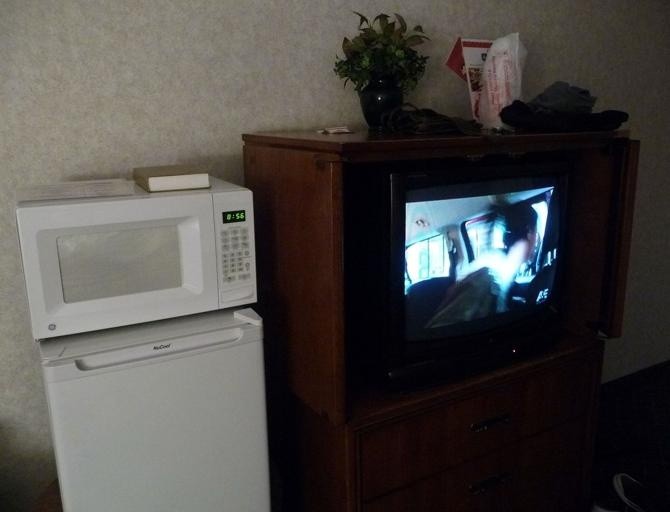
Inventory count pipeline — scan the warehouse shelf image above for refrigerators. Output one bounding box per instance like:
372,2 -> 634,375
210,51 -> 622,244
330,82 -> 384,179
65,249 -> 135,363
33,307 -> 274,512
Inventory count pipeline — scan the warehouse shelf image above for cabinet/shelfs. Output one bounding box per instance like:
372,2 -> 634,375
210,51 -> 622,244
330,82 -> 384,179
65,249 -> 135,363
267,331 -> 607,512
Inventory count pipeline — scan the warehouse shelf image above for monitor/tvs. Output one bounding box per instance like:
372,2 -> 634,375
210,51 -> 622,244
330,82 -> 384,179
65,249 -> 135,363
376,164 -> 570,393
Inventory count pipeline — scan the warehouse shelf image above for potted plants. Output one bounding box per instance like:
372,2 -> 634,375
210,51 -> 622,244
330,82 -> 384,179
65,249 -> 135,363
331,9 -> 430,134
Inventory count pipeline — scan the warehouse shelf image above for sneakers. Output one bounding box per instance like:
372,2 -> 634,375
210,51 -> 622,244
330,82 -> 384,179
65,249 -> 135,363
594,473 -> 645,512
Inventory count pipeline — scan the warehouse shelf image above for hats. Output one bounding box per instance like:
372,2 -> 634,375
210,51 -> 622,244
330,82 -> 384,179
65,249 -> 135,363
498,81 -> 629,131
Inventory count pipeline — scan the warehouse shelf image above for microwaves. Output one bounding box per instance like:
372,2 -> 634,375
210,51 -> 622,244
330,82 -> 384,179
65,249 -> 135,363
16,176 -> 257,343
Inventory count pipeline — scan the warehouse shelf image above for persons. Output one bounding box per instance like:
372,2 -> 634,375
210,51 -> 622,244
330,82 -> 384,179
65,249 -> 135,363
425,198 -> 538,329
